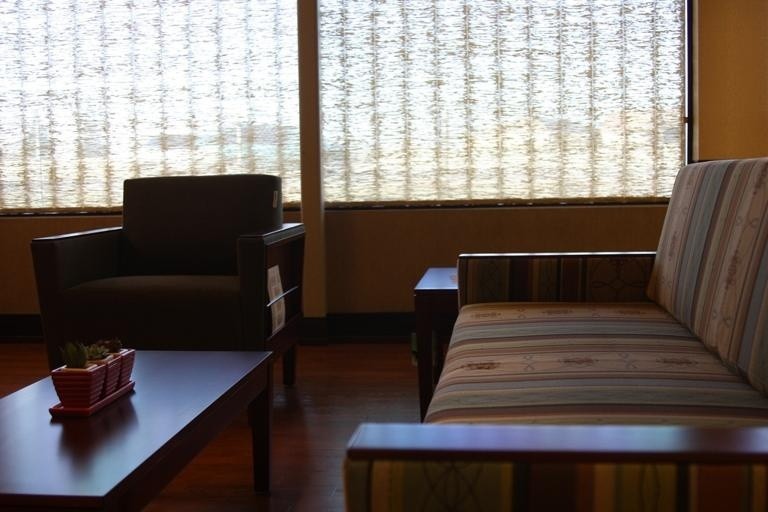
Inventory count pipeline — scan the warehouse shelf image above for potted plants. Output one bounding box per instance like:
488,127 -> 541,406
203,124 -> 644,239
106,337 -> 135,390
51,341 -> 106,406
84,341 -> 122,397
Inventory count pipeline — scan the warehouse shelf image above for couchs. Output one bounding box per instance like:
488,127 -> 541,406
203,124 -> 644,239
343,159 -> 768,511
29,176 -> 307,387
